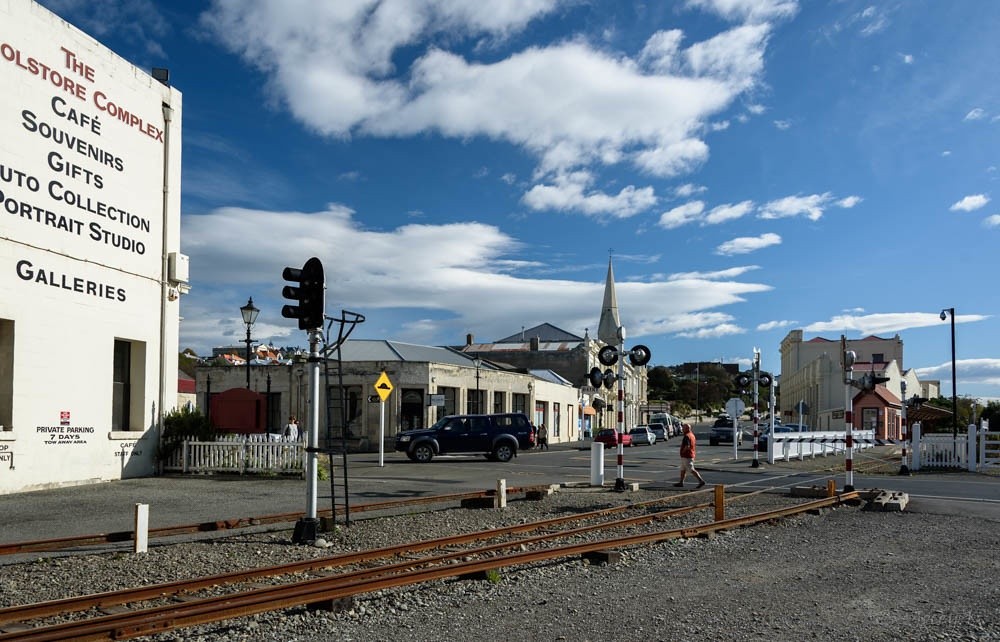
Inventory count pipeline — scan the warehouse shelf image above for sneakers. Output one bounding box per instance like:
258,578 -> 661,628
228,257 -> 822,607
673,481 -> 683,487
696,481 -> 705,489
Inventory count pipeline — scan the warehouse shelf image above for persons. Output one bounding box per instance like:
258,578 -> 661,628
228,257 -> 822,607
531,420 -> 537,437
536,425 -> 542,447
281,415 -> 299,454
293,420 -> 304,455
539,424 -> 550,450
673,423 -> 706,490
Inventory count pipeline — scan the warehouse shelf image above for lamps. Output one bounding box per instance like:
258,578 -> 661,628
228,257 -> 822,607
152,68 -> 169,86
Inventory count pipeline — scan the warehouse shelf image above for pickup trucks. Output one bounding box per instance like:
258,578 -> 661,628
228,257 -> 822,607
595,428 -> 633,448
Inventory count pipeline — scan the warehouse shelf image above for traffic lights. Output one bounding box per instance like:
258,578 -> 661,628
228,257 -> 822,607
280,266 -> 306,320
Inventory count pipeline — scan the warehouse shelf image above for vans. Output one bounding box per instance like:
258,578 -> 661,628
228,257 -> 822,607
649,413 -> 674,437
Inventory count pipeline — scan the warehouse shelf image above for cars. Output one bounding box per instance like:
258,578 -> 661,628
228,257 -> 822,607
709,417 -> 744,446
758,416 -> 808,449
670,415 -> 685,436
392,413 -> 535,463
628,427 -> 657,446
640,423 -> 669,441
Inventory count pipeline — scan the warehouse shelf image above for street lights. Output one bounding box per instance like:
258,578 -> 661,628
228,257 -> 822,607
238,294 -> 261,388
470,352 -> 484,415
940,306 -> 960,466
